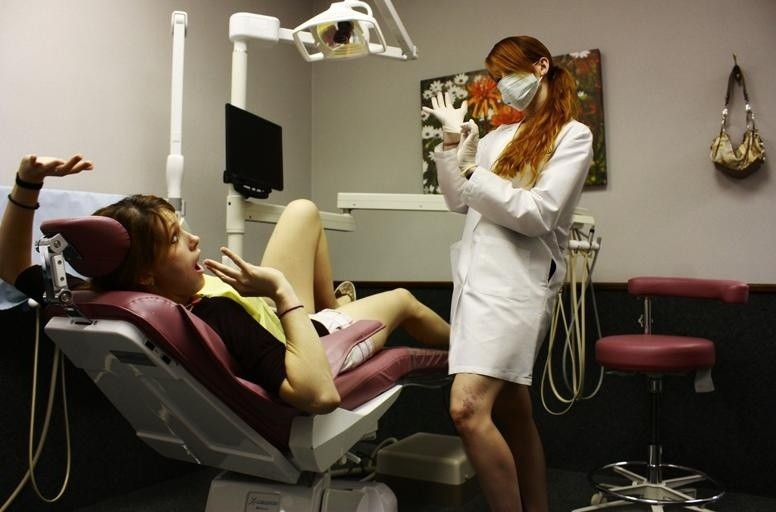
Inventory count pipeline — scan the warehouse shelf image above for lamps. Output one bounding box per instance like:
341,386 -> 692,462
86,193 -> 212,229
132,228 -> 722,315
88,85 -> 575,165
289,0 -> 420,67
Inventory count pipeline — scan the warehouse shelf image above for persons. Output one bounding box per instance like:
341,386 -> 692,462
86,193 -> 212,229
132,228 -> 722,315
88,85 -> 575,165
419,34 -> 596,511
0,154 -> 451,416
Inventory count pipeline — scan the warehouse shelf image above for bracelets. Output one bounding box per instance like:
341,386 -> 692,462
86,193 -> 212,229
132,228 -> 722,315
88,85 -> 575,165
14,172 -> 43,190
6,193 -> 39,212
275,303 -> 304,319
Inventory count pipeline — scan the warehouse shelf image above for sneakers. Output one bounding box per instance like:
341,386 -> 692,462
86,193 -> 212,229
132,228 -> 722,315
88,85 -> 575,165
335,281 -> 356,307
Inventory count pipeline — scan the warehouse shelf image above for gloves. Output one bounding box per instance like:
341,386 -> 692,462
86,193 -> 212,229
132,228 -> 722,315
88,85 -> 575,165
423,92 -> 469,132
458,119 -> 479,174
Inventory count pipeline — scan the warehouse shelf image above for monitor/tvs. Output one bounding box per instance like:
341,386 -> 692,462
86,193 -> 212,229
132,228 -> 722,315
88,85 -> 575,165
224,102 -> 284,200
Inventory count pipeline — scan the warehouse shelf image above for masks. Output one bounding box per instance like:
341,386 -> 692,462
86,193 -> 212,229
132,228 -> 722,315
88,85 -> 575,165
496,63 -> 543,111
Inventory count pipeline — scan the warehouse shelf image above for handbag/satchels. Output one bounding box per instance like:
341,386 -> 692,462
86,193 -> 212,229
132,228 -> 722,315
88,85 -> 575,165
709,129 -> 766,178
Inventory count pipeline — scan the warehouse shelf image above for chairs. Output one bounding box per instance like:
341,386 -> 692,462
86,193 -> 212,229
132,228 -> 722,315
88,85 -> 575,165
570,275 -> 750,512
33,215 -> 451,512
